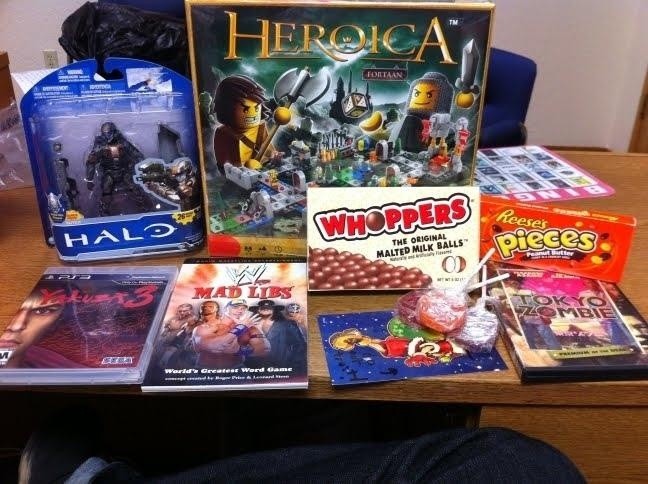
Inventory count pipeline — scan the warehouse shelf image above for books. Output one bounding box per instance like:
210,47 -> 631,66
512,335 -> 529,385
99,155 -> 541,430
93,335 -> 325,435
485,259 -> 648,384
0,266 -> 180,386
139,255 -> 308,392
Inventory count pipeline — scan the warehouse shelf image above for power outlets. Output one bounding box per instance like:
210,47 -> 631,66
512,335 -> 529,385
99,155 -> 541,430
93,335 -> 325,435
42,50 -> 59,69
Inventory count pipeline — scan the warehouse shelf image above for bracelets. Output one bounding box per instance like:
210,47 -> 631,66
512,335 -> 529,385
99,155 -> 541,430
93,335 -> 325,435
227,323 -> 248,338
233,344 -> 254,357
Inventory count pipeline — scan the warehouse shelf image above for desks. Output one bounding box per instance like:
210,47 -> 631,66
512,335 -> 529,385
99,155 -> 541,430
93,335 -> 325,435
0,149 -> 647,484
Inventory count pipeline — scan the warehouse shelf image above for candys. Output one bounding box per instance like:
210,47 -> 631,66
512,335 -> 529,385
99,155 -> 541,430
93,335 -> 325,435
396,246 -> 512,354
310,243 -> 431,291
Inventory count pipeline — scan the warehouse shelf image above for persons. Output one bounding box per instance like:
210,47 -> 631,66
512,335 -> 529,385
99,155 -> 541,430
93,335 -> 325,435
173,300 -> 222,366
192,297 -> 271,370
18,425 -> 588,484
152,303 -> 198,370
249,299 -> 307,367
1,285 -> 92,368
287,303 -> 307,340
